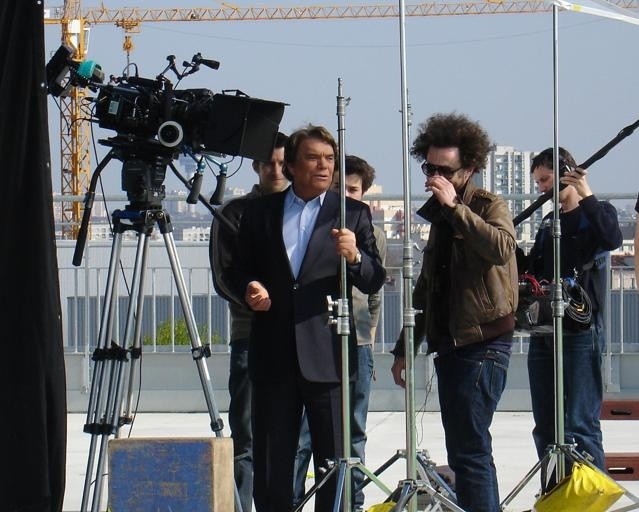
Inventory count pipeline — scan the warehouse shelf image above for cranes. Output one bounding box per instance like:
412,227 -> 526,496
44,0 -> 567,241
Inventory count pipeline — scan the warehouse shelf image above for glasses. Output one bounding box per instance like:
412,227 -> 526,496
421,160 -> 466,180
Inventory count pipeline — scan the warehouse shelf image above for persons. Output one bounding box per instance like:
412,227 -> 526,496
510,144 -> 623,497
388,108 -> 521,512
222,125 -> 385,511
288,156 -> 388,511
209,128 -> 291,512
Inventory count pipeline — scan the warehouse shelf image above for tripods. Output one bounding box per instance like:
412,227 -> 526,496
79,209 -> 243,512
293,78 -> 406,512
355,0 -> 465,512
500,1 -> 639,512
